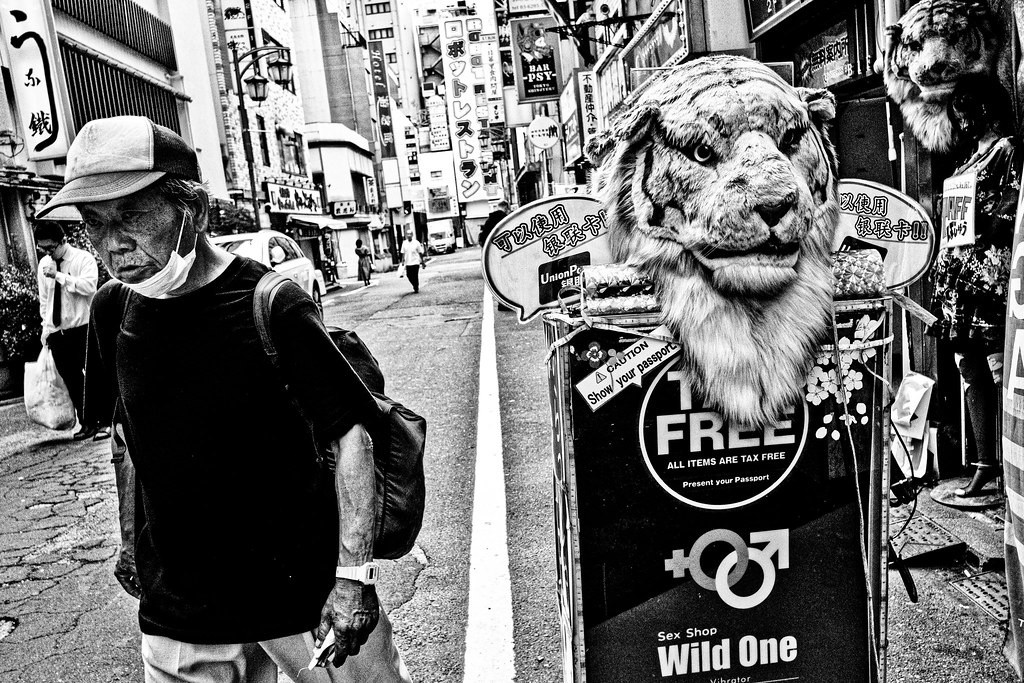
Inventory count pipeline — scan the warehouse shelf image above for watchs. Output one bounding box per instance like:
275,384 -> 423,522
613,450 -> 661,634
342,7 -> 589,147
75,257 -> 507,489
336,562 -> 379,585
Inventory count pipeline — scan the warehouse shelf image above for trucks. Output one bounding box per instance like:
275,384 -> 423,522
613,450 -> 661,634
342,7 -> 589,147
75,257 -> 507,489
426,216 -> 457,256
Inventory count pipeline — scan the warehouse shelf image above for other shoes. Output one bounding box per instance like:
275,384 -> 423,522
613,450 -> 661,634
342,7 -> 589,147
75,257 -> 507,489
73,425 -> 112,441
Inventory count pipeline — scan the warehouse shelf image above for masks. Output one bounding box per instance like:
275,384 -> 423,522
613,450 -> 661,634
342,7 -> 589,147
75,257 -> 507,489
105,210 -> 198,301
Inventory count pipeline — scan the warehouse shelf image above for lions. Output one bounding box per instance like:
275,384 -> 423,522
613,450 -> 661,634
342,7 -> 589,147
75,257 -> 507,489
584,53 -> 842,435
882,0 -> 1006,154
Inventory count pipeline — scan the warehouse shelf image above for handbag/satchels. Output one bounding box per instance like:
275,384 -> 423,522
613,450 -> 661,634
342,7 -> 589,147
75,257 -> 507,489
24,344 -> 77,432
397,263 -> 406,279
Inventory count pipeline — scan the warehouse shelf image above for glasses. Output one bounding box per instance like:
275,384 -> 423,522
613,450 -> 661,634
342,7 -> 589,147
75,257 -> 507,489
36,242 -> 61,253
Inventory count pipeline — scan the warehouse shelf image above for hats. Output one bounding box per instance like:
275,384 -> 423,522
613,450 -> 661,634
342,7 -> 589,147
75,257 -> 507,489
35,114 -> 201,218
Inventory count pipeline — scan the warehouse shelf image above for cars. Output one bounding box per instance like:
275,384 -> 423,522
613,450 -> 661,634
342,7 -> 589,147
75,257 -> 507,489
209,229 -> 327,322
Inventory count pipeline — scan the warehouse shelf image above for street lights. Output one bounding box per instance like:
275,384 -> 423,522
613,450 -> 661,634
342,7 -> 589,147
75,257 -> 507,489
228,35 -> 293,232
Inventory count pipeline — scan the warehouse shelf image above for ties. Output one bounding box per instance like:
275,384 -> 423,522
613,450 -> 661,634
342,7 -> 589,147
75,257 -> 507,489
52,257 -> 64,327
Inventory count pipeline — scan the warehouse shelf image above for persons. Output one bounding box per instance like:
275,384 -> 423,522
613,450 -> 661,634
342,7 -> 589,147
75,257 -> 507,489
924,86 -> 1021,498
400,230 -> 426,294
35,115 -> 413,683
34,222 -> 113,442
355,238 -> 373,286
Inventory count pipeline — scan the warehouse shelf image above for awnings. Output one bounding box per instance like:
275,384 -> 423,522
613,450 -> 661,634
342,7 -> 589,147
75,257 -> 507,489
290,215 -> 347,230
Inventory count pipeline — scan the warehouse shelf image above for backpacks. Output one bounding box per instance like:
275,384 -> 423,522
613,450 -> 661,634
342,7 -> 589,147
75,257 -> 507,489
113,269 -> 426,561
